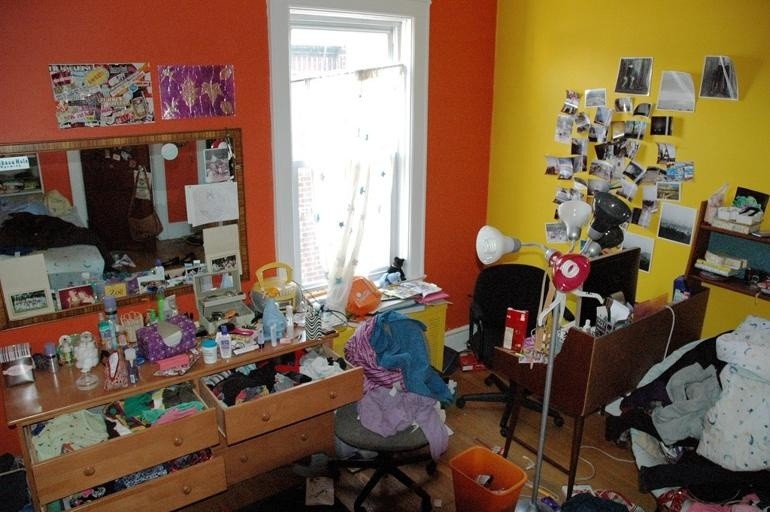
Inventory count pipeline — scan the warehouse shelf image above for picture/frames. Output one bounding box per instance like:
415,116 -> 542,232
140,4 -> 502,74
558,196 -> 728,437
1,152 -> 44,195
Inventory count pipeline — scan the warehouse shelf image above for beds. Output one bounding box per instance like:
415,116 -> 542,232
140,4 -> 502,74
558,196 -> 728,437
0,195 -> 105,289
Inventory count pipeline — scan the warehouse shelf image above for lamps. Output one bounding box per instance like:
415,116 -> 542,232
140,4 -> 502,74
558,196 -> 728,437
546,248 -> 589,292
476,225 -> 547,264
161,142 -> 187,161
589,226 -> 624,256
559,200 -> 593,255
587,191 -> 632,240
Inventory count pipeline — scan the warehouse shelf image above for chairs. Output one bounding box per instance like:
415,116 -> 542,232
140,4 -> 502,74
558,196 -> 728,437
457,263 -> 563,437
324,312 -> 438,512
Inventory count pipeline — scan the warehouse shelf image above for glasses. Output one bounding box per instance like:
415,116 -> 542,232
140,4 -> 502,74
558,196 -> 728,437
231,341 -> 245,351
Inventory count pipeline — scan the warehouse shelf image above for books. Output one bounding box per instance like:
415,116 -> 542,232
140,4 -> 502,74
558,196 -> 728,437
695,249 -> 747,278
711,218 -> 760,236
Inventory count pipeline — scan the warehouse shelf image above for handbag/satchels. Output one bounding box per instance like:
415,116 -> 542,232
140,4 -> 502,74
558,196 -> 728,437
126,164 -> 164,242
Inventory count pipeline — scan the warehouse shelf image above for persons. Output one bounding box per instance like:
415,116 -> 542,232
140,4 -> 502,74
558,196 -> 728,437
143,281 -> 158,293
67,289 -> 80,308
79,291 -> 94,304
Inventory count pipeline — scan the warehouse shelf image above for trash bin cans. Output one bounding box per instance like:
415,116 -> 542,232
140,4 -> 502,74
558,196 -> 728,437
449,445 -> 527,512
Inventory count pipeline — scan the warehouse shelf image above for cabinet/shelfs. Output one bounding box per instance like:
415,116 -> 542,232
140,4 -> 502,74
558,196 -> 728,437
7,332 -> 363,512
682,200 -> 770,337
310,301 -> 446,374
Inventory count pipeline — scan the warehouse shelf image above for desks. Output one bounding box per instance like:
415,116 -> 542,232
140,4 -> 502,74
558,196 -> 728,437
492,279 -> 710,501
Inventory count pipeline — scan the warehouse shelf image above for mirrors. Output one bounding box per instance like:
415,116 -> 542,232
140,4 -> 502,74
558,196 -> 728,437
0,128 -> 251,333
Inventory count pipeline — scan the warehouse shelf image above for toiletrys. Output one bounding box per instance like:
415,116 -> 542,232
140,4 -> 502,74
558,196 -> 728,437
200,340 -> 217,364
257,326 -> 265,349
233,344 -> 259,355
286,306 -> 294,338
271,324 -> 277,347
219,325 -> 232,359
